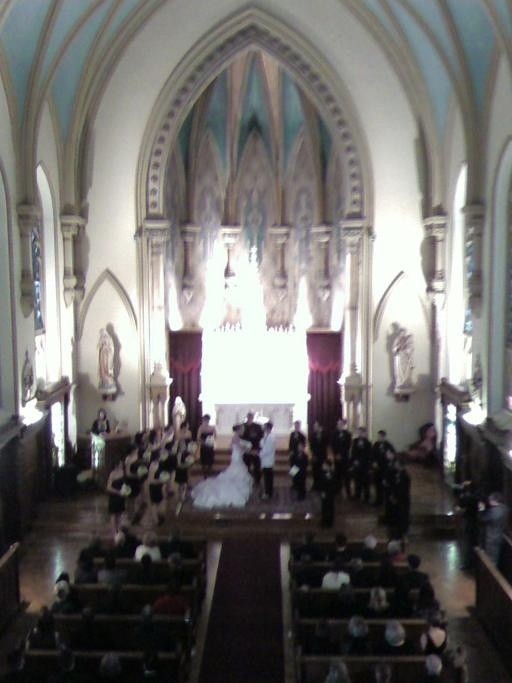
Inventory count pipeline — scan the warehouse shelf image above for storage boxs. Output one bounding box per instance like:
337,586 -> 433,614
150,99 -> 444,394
288,534 -> 469,682
22,537 -> 206,683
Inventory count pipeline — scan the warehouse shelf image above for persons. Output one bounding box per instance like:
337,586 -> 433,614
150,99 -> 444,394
308,420 -> 329,490
10,527 -> 197,681
259,423 -> 275,499
90,410 -> 111,436
96,328 -> 115,389
392,328 -> 417,391
107,420 -> 201,535
240,413 -> 265,488
373,431 -> 411,534
190,424 -> 254,510
291,531 -> 468,681
319,459 -> 341,528
197,415 -> 217,480
289,441 -> 308,501
288,421 -> 306,488
351,428 -> 373,503
330,419 -> 352,499
